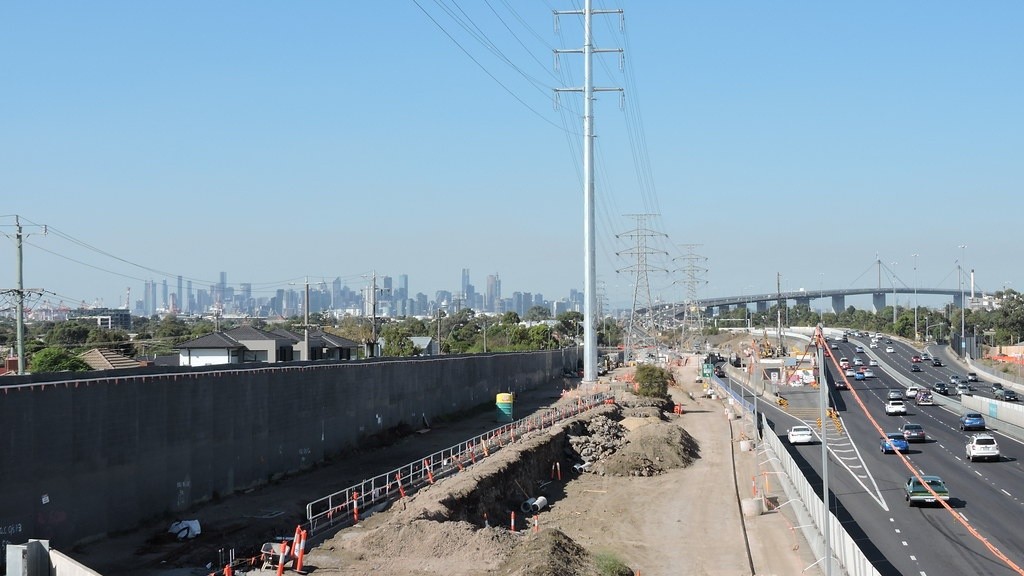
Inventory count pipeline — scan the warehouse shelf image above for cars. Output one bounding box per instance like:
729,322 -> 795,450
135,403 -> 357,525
931,358 -> 941,367
819,329 -> 896,381
884,400 -> 907,416
959,411 -> 986,431
786,425 -> 814,445
880,432 -> 910,455
887,389 -> 903,401
906,387 -> 919,399
920,352 -> 929,360
900,423 -> 925,443
910,364 -> 920,373
916,389 -> 934,406
911,355 -> 921,363
949,371 -> 1019,403
903,475 -> 950,508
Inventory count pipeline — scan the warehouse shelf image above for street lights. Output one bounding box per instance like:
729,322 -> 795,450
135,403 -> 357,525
957,245 -> 969,337
891,261 -> 899,325
819,273 -> 825,320
912,254 -> 920,342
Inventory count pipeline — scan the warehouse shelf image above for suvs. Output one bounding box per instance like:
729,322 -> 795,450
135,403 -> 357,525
964,432 -> 1001,463
933,382 -> 949,396
